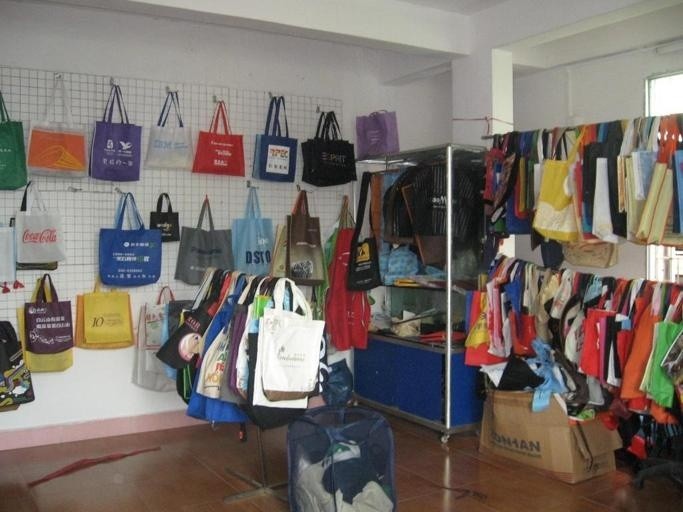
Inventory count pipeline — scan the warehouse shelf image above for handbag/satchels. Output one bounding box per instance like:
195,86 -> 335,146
0,212 -> 73,412
174,196 -> 234,285
464,275 -> 682,425
1,91 -> 28,190
150,192 -> 180,243
191,101 -> 245,177
269,223 -> 287,277
143,93 -> 194,170
561,244 -> 617,268
28,75 -> 86,172
252,96 -> 297,182
74,291 -> 134,350
131,296 -> 327,430
99,192 -> 162,286
327,195 -> 381,291
355,112 -> 396,160
471,143 -> 681,247
230,189 -> 274,277
286,192 -> 326,286
88,84 -> 142,182
300,112 -> 358,187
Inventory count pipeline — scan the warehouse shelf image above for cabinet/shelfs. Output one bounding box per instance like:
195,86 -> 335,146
348,143 -> 491,444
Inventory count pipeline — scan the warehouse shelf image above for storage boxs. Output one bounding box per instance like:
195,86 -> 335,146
479,375 -> 625,485
